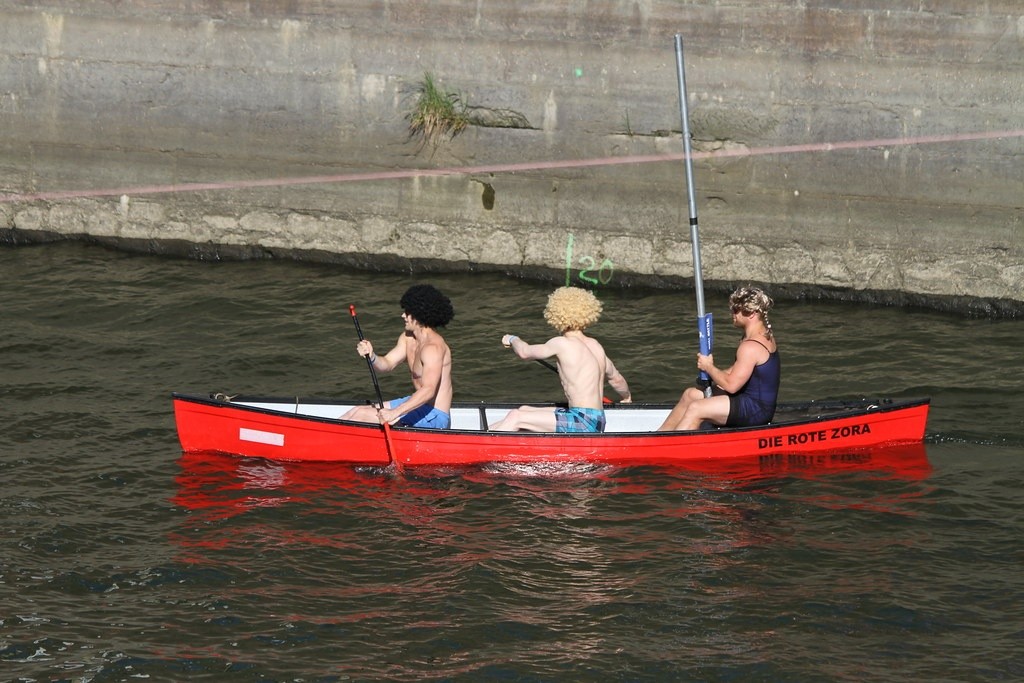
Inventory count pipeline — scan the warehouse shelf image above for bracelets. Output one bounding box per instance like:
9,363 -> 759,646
372,355 -> 377,363
509,336 -> 518,344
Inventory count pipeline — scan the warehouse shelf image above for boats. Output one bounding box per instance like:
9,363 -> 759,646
172,392 -> 932,466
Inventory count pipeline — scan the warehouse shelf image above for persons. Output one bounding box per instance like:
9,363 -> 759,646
336,284 -> 456,429
489,285 -> 631,432
657,286 -> 780,431
